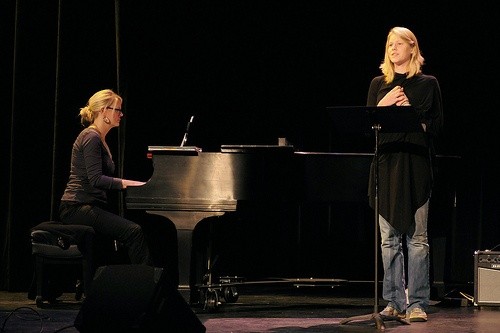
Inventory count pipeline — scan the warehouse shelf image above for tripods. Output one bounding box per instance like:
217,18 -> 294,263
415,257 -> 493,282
325,104 -> 417,331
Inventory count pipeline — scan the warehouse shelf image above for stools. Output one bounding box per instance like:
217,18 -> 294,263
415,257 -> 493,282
31,242 -> 87,310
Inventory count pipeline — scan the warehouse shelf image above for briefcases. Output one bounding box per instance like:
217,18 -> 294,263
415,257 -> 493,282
473,244 -> 500,307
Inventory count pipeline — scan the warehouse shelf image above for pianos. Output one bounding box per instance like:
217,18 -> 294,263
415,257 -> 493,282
122,143 -> 298,311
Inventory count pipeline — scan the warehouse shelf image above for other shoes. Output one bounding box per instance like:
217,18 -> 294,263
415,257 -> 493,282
408,307 -> 428,322
379,306 -> 407,318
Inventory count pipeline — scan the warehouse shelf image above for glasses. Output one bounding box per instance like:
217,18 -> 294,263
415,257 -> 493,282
106,106 -> 122,116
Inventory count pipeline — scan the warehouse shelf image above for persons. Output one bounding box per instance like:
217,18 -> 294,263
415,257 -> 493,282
367,26 -> 442,322
60,89 -> 180,290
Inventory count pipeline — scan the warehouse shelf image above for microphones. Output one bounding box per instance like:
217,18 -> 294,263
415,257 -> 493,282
181,114 -> 193,147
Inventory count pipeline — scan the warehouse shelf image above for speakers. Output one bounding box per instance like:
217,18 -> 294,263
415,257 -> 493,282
72,263 -> 206,333
473,251 -> 500,310
